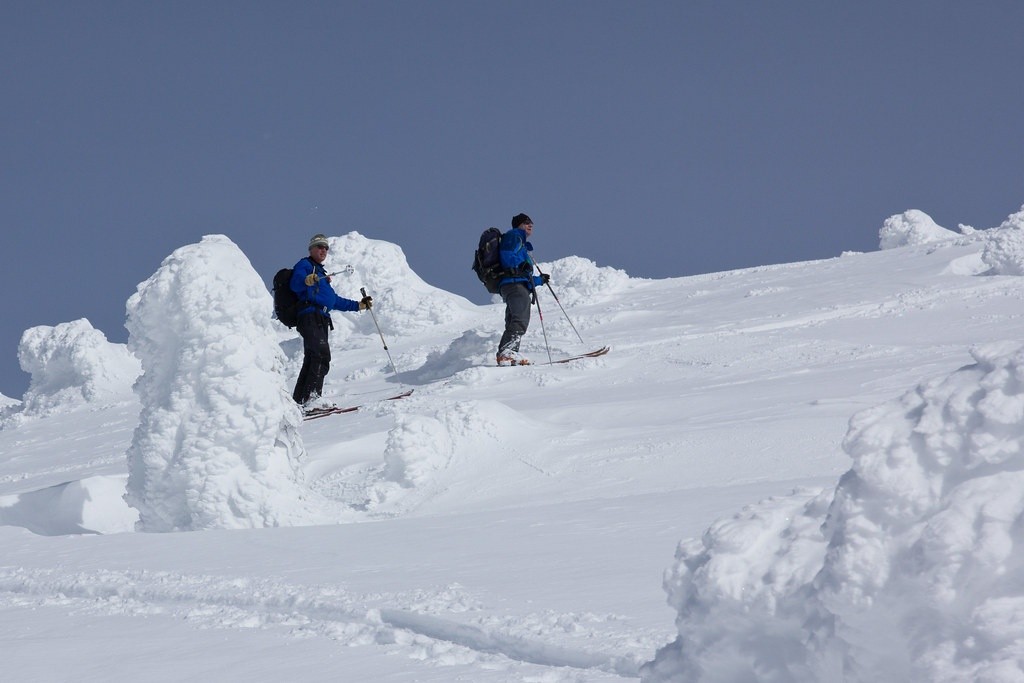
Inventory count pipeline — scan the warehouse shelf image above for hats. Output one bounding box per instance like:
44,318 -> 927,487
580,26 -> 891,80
512,213 -> 529,229
308,233 -> 328,250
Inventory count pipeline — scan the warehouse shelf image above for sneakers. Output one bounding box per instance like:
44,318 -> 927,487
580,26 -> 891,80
496,351 -> 531,366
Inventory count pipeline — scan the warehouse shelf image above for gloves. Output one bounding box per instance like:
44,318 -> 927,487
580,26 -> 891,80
540,274 -> 550,286
358,296 -> 373,310
518,261 -> 533,274
304,274 -> 319,286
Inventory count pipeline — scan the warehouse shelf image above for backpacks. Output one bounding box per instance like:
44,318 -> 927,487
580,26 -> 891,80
272,268 -> 296,329
472,227 -> 501,294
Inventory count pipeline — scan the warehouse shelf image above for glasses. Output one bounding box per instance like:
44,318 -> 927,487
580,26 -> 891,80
318,245 -> 329,250
522,219 -> 534,224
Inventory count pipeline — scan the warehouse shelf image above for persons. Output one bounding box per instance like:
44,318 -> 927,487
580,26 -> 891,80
291,234 -> 373,412
497,213 -> 550,366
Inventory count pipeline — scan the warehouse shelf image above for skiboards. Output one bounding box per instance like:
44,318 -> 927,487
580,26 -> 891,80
303,388 -> 414,422
477,344 -> 612,367
